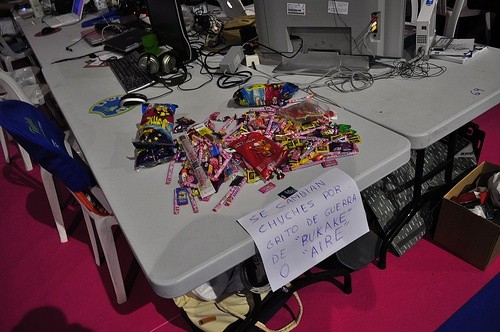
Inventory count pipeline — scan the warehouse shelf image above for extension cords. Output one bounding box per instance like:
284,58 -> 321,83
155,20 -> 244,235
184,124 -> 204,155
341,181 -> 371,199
243,43 -> 260,66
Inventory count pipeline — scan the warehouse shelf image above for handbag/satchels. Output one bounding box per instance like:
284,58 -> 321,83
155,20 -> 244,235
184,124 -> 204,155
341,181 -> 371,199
223,15 -> 259,47
173,257 -> 302,332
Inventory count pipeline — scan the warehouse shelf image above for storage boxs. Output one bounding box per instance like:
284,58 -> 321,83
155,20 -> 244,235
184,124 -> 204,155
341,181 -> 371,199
435,160 -> 500,272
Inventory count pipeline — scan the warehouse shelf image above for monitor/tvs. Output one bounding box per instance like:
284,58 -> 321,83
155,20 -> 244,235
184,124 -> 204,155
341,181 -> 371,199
144,0 -> 199,66
253,0 -> 406,77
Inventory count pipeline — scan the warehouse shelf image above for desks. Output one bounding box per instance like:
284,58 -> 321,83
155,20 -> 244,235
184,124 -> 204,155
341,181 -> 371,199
10,7 -> 110,67
225,34 -> 500,269
41,45 -> 411,332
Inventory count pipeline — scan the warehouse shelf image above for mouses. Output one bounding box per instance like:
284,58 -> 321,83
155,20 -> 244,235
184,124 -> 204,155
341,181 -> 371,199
119,93 -> 147,106
42,27 -> 55,34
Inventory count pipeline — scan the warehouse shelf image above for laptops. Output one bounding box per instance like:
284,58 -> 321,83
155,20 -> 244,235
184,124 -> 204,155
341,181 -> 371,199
218,0 -> 256,17
42,0 -> 84,28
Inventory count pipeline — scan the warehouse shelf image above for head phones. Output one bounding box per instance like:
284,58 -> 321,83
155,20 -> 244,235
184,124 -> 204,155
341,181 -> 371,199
137,52 -> 187,86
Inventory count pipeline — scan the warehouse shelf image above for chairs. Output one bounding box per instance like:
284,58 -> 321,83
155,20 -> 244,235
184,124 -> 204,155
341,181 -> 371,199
0,37 -> 126,303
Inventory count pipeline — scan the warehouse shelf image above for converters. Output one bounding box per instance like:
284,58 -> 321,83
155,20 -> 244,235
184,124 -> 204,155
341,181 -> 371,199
220,46 -> 244,73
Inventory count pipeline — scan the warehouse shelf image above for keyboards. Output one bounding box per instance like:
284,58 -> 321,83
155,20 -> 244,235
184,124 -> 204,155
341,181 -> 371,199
107,50 -> 162,93
